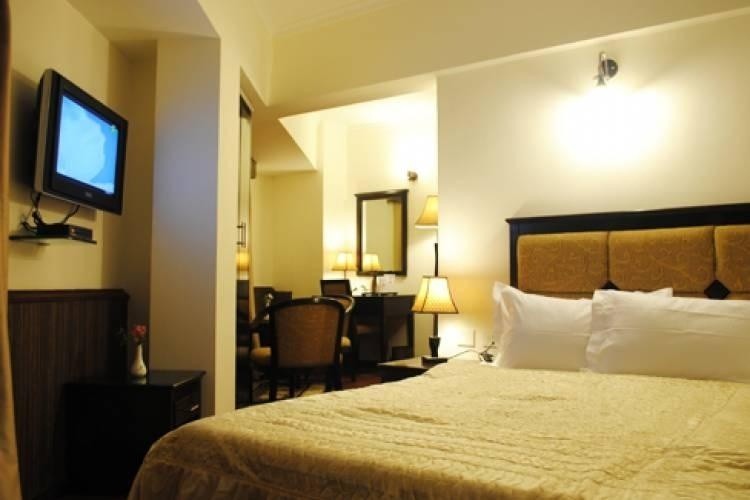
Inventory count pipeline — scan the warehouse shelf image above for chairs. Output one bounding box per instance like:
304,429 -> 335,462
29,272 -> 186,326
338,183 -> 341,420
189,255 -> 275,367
312,294 -> 356,392
244,295 -> 346,408
320,279 -> 380,368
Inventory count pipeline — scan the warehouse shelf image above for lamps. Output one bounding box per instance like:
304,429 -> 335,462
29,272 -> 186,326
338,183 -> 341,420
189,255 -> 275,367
411,241 -> 460,366
363,254 -> 383,270
331,253 -> 357,279
415,196 -> 439,277
591,49 -> 620,88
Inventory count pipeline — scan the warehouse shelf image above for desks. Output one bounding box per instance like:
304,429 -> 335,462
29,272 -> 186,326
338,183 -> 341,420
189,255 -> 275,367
352,294 -> 416,368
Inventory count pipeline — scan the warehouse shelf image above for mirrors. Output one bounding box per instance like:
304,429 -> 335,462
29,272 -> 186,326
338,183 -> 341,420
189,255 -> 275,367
354,189 -> 409,277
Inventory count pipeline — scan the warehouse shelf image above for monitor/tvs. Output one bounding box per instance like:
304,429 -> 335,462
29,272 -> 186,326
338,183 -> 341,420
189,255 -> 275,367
32,69 -> 128,215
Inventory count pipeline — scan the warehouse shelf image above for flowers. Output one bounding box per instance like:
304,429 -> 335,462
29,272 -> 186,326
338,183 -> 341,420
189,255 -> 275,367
118,324 -> 151,348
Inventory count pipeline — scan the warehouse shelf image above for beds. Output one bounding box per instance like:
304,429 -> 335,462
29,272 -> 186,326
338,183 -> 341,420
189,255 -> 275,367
126,200 -> 748,500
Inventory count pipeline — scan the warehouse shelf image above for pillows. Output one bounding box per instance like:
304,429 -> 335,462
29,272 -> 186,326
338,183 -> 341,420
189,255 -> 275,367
585,287 -> 748,383
490,277 -> 673,374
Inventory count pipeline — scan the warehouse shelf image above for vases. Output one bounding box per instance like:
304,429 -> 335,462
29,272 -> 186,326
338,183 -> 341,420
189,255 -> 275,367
129,343 -> 149,378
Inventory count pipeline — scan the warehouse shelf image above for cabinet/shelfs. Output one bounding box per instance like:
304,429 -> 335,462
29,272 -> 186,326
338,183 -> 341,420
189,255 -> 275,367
62,368 -> 206,500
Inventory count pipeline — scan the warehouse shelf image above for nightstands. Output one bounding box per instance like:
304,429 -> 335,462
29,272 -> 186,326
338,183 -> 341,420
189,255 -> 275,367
378,353 -> 449,383
